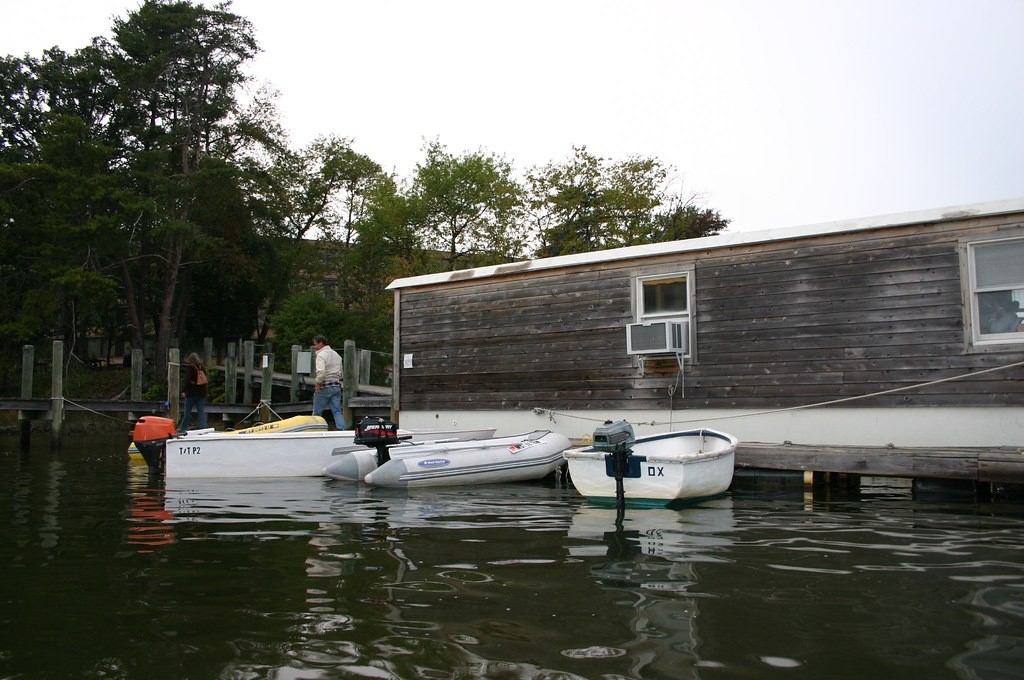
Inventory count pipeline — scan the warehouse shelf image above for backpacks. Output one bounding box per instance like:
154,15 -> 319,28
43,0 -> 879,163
191,363 -> 208,385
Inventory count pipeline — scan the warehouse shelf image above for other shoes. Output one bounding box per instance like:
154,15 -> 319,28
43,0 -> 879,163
176,429 -> 186,432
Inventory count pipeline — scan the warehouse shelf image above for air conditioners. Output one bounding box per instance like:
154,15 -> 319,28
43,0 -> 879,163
624,321 -> 687,355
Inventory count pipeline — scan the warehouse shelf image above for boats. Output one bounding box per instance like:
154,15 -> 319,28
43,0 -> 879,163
561,428 -> 738,500
320,416 -> 574,490
127,414 -> 332,459
163,426 -> 503,477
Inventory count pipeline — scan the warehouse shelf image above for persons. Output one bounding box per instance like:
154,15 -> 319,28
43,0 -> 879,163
178,352 -> 207,431
312,334 -> 347,430
